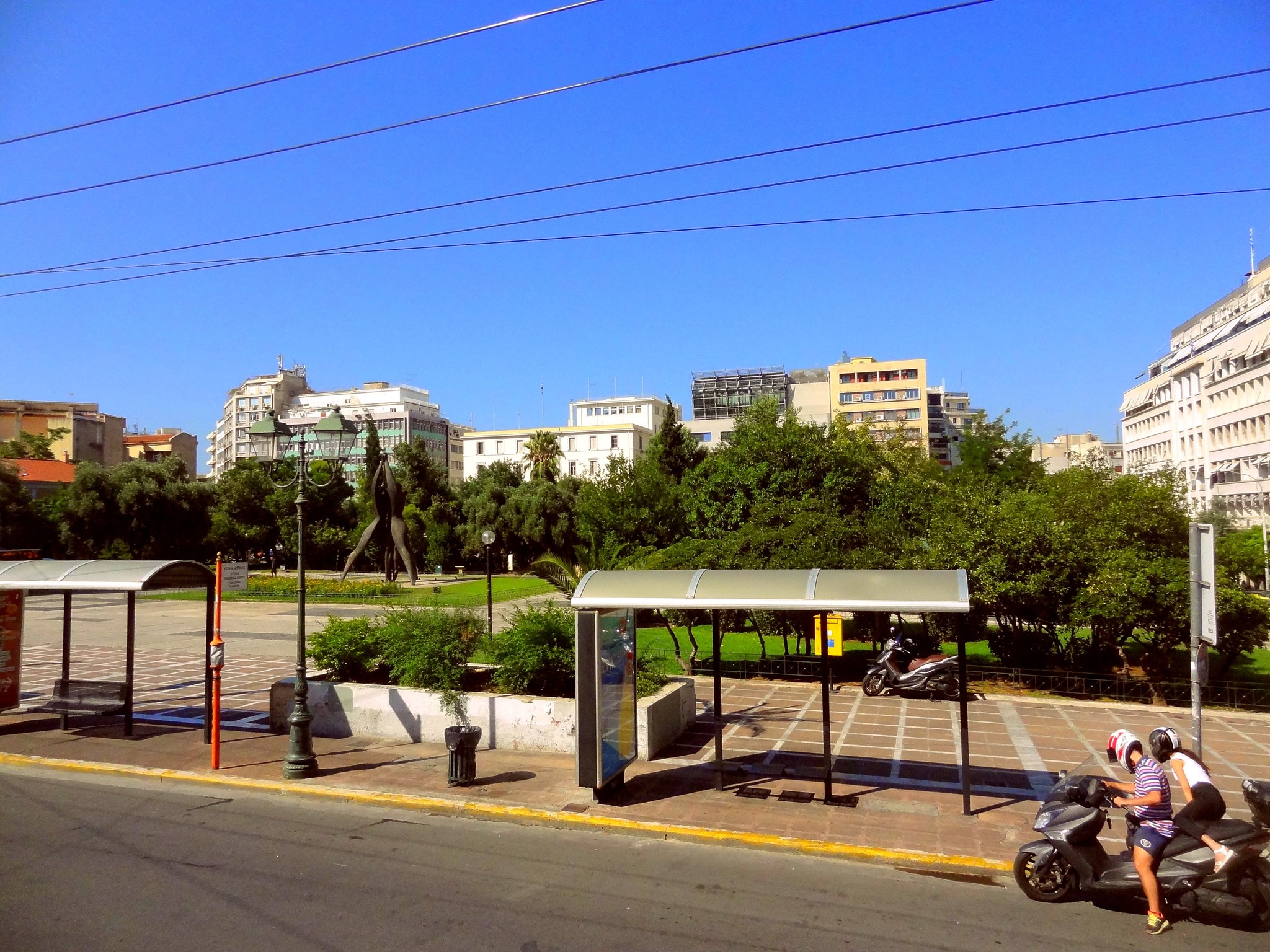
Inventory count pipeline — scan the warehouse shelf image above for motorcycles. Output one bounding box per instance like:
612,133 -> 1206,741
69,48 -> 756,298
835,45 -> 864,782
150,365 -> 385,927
1014,771 -> 1270,932
862,626 -> 962,701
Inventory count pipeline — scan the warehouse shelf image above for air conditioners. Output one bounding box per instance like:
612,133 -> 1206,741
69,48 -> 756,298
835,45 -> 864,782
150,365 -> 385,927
304,449 -> 307,454
340,447 -> 344,451
379,446 -> 384,451
250,404 -> 255,408
277,451 -> 282,455
362,407 -> 367,411
328,448 -> 332,451
307,449 -> 313,453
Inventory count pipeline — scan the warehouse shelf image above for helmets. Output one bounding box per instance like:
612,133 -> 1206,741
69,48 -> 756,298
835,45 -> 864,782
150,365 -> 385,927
1107,729 -> 1143,774
1148,727 -> 1182,764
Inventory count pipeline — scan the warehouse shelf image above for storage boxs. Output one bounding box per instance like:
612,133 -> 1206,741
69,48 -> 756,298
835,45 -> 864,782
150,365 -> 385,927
1241,778 -> 1270,829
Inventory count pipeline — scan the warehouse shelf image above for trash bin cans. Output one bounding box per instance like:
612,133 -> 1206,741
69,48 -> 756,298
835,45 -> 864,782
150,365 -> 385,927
444,726 -> 482,787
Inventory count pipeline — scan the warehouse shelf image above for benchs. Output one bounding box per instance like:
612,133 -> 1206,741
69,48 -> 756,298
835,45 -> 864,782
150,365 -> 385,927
27,678 -> 130,730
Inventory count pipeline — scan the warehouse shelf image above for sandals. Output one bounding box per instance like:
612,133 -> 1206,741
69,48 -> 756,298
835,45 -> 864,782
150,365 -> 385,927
1213,844 -> 1234,874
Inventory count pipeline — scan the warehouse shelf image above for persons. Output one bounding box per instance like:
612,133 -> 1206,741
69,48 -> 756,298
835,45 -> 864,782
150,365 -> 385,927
1100,728 -> 1176,935
270,557 -> 277,578
1149,726 -> 1235,875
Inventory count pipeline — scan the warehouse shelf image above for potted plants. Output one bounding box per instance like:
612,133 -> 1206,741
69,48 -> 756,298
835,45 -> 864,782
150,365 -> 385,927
427,662 -> 482,787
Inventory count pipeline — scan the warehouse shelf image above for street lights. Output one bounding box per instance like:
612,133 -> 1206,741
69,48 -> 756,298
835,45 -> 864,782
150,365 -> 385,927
480,528 -> 497,643
423,532 -> 428,565
243,402 -> 358,780
244,533 -> 250,561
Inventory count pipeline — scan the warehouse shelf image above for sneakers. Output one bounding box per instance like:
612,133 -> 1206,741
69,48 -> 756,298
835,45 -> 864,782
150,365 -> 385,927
1145,910 -> 1169,935
